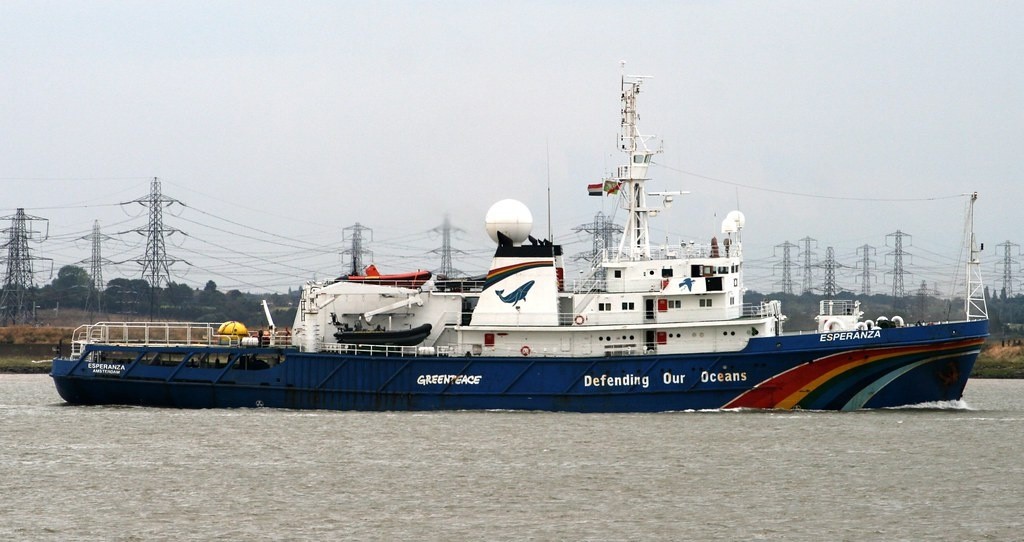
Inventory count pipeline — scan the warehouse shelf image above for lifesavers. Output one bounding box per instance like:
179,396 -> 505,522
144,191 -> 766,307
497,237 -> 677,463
853,322 -> 867,331
575,316 -> 584,325
865,320 -> 874,329
891,316 -> 904,326
823,318 -> 847,333
520,346 -> 531,357
876,316 -> 888,325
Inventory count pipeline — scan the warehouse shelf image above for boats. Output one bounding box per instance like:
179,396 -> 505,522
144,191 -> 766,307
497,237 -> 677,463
47,59 -> 991,411
